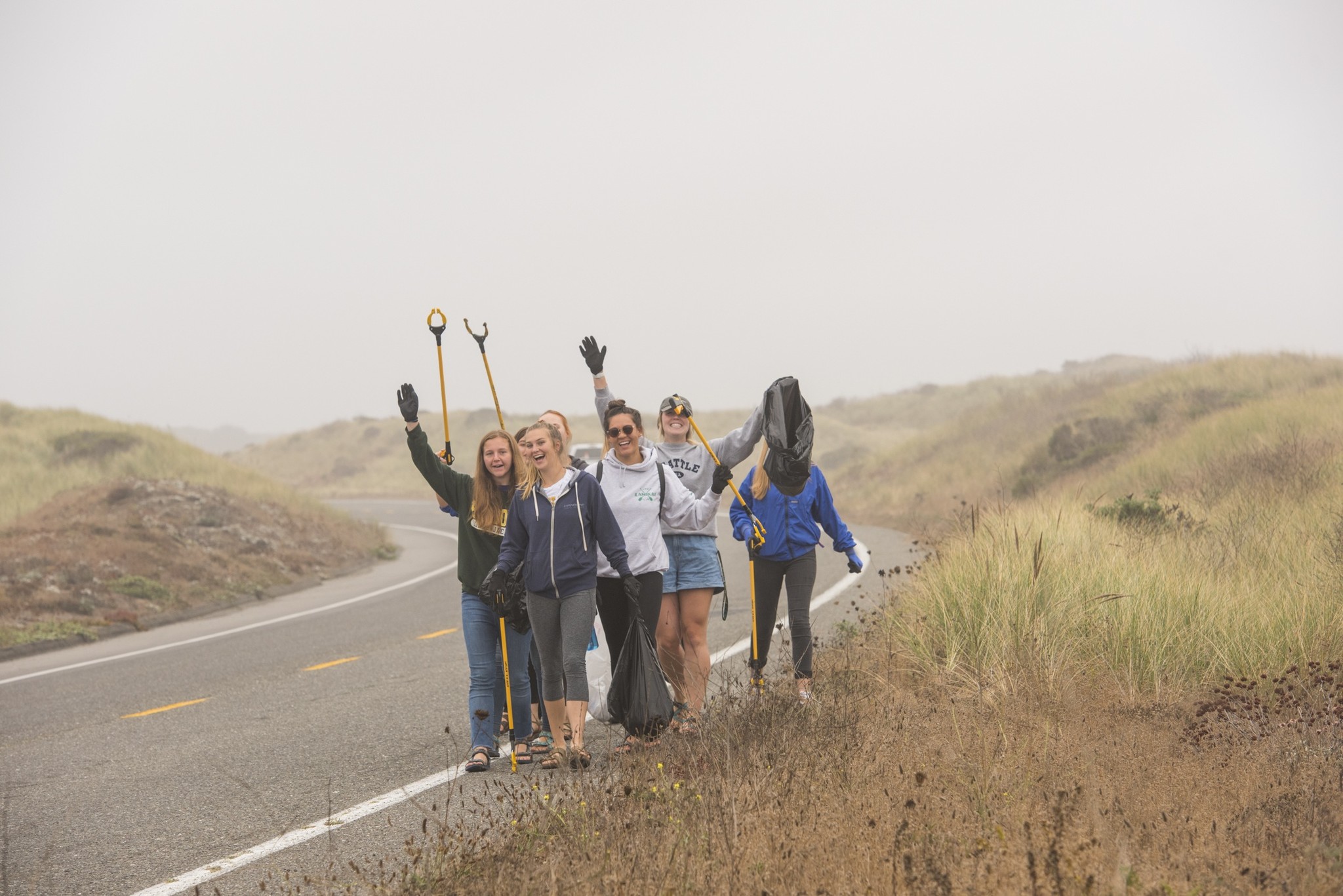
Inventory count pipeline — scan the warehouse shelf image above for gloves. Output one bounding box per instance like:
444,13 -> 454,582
488,570 -> 508,595
740,524 -> 762,559
711,465 -> 733,494
846,549 -> 863,573
397,382 -> 419,422
623,574 -> 643,599
579,335 -> 607,375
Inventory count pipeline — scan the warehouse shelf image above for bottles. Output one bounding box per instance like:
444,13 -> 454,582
587,628 -> 599,650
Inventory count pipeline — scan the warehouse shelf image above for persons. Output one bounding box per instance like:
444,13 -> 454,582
514,408 -> 589,756
397,384 -> 535,770
730,463 -> 861,702
480,420 -> 641,770
582,398 -> 731,676
577,335 -> 766,733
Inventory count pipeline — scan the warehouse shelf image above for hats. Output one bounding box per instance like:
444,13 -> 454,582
660,395 -> 693,415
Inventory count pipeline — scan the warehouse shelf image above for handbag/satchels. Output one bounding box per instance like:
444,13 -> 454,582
477,556 -> 532,636
585,603 -> 614,722
760,375 -> 814,497
607,592 -> 675,743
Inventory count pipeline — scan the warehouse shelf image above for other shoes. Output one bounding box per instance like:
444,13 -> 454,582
798,690 -> 815,705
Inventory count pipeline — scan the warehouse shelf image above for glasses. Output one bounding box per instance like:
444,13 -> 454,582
606,424 -> 636,438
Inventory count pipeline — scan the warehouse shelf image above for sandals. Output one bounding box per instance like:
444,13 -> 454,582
570,749 -> 592,769
679,717 -> 704,736
465,748 -> 491,772
670,701 -> 689,730
513,739 -> 533,764
487,746 -> 500,758
540,747 -> 570,768
527,720 -> 542,742
613,733 -> 643,755
500,714 -> 509,734
531,731 -> 555,754
564,722 -> 572,740
